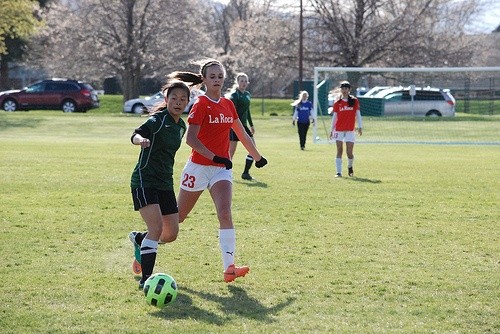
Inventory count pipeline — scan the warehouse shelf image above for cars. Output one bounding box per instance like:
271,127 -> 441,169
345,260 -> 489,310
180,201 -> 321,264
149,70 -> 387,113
123,85 -> 206,114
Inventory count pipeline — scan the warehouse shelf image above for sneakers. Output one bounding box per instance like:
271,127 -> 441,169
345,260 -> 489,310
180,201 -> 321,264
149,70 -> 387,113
132,257 -> 142,274
223,264 -> 250,283
138,281 -> 144,290
241,172 -> 254,181
130,231 -> 142,264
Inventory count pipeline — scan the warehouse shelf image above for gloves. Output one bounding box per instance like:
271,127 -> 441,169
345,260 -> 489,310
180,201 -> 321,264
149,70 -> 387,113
256,157 -> 267,169
213,155 -> 233,170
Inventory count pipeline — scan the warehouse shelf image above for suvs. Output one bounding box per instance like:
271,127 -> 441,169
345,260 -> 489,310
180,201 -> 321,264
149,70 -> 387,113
326,85 -> 456,121
0,76 -> 100,113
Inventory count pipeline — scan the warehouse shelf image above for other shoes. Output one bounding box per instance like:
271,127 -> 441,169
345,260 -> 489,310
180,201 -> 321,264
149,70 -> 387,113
336,173 -> 341,177
348,166 -> 354,177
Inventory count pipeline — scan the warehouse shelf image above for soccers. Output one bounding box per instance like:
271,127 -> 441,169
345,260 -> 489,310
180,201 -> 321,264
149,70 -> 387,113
143,273 -> 178,308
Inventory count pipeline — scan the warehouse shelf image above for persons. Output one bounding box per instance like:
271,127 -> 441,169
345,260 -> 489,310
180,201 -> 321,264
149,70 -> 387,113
290,91 -> 316,150
128,82 -> 190,290
328,80 -> 362,178
133,58 -> 249,282
224,73 -> 256,181
